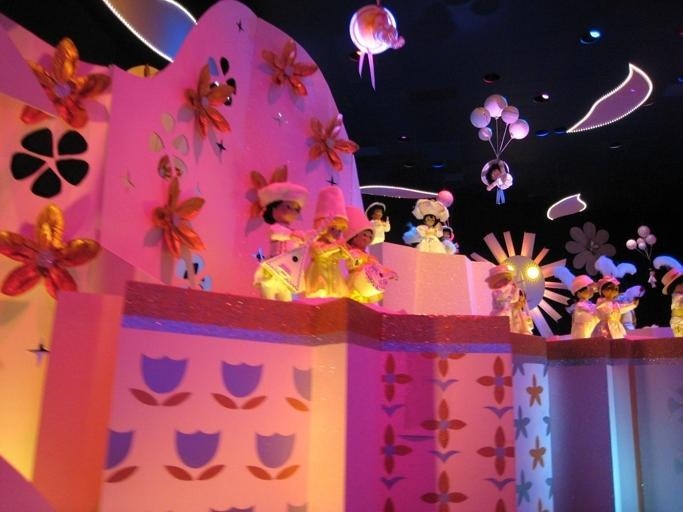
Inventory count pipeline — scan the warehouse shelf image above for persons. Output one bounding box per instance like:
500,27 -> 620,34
486,159 -> 506,205
411,197 -> 459,256
254,182 -> 397,304
510,269 -> 683,340
251,156 -> 683,343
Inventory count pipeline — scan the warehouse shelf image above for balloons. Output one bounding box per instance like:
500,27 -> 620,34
626,225 -> 655,250
477,127 -> 492,142
484,94 -> 507,120
501,105 -> 519,123
508,118 -> 530,141
470,107 -> 491,128
